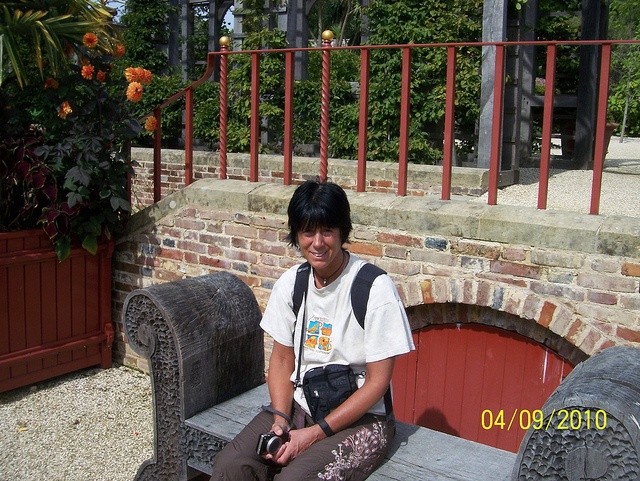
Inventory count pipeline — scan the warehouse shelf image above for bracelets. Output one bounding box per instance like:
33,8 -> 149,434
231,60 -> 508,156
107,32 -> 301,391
316,417 -> 336,438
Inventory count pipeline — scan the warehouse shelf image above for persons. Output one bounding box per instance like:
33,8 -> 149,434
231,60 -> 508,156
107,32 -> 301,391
209,181 -> 415,481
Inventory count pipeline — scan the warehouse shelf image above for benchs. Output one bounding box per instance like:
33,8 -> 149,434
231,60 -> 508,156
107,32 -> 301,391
121,270 -> 640,480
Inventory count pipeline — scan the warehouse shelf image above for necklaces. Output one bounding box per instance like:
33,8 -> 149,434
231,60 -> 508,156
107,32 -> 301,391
310,248 -> 346,286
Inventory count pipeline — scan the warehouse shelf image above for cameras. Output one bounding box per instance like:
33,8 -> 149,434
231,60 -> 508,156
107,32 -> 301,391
254,431 -> 291,461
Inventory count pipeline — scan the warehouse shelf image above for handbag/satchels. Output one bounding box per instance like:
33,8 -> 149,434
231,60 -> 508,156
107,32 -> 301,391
296,262 -> 366,424
